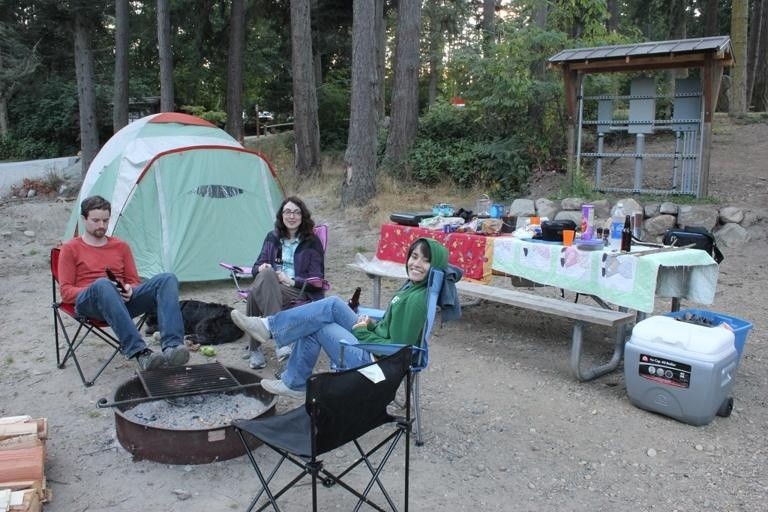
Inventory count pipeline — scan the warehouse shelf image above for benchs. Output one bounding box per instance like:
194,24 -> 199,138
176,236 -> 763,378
364,259 -> 635,382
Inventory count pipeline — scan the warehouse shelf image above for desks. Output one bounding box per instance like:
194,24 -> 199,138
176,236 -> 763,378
381,217 -> 706,344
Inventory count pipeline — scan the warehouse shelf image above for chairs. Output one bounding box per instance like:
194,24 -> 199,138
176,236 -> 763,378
329,268 -> 445,447
229,344 -> 412,512
49,249 -> 153,387
220,224 -> 330,354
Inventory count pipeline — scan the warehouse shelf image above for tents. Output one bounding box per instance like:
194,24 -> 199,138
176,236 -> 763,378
64,112 -> 288,284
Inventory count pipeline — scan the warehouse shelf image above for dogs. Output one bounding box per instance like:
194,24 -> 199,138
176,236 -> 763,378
144,300 -> 245,345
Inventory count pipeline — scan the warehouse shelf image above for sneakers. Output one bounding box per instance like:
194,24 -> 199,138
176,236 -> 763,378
274,344 -> 292,362
249,351 -> 267,369
137,350 -> 165,370
163,346 -> 190,369
262,380 -> 307,398
232,310 -> 272,344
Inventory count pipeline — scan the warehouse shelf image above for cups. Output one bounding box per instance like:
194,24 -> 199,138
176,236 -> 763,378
562,230 -> 574,246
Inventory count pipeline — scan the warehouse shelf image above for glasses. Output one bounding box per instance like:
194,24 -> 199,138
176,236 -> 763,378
282,209 -> 302,216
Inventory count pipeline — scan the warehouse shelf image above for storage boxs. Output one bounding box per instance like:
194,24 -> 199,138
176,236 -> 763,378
623,307 -> 753,427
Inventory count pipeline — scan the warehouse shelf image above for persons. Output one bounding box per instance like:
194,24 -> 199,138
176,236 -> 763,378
58,195 -> 191,370
245,196 -> 324,369
230,236 -> 449,400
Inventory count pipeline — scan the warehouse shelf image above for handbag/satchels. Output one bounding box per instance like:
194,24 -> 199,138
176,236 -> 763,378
663,226 -> 723,264
541,219 -> 576,242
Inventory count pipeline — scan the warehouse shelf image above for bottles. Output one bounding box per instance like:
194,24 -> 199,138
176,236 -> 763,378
596,226 -> 603,239
347,286 -> 362,312
273,246 -> 284,273
602,228 -> 609,246
104,267 -> 127,295
580,204 -> 595,240
610,202 -> 625,250
620,215 -> 631,252
